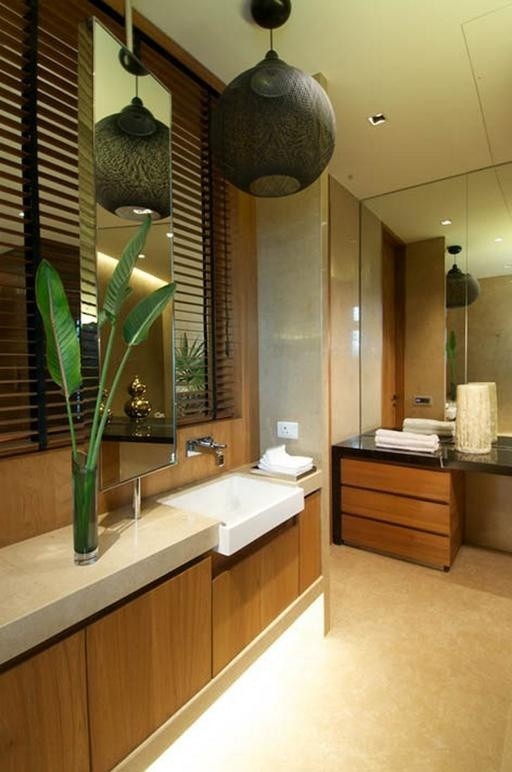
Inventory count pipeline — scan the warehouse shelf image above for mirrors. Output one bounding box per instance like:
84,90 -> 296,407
358,161 -> 512,448
92,14 -> 179,494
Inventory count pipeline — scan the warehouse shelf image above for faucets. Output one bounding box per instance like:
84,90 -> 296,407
186,436 -> 227,468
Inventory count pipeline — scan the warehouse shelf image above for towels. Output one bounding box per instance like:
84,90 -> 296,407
375,429 -> 440,453
258,445 -> 313,476
403,418 -> 455,437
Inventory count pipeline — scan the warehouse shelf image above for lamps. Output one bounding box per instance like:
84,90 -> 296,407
96,46 -> 171,222
212,0 -> 336,197
445,246 -> 479,307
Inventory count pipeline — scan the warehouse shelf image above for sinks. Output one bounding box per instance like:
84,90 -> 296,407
155,471 -> 306,558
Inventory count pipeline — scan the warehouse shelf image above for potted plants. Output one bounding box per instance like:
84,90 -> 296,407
34,214 -> 182,566
445,331 -> 459,404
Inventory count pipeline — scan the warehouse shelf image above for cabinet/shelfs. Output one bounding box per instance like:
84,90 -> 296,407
296,488 -> 324,596
86,555 -> 212,771
212,524 -> 299,680
341,459 -> 467,570
1,628 -> 91,772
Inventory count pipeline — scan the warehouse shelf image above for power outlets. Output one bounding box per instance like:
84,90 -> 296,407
277,421 -> 299,439
